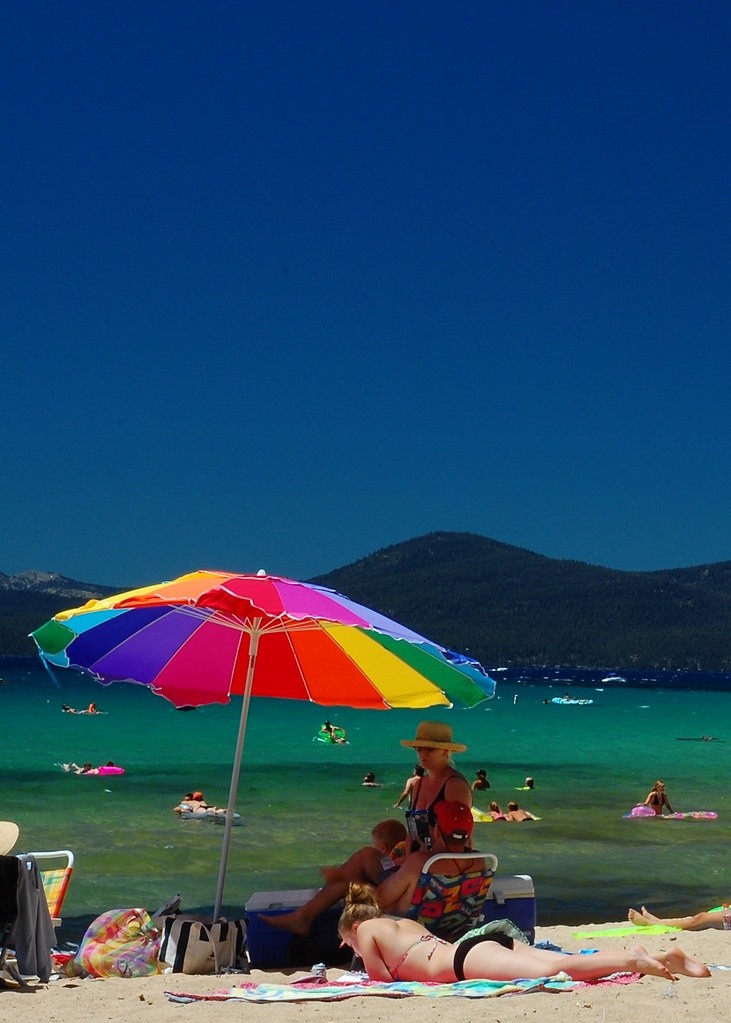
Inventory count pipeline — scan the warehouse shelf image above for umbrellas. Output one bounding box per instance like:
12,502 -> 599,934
24,566 -> 500,923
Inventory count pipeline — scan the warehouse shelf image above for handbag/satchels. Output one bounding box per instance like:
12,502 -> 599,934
155,916 -> 250,975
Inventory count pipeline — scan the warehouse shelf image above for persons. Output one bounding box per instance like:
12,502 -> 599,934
471,769 -> 491,791
63,702 -> 100,715
172,793 -> 227,815
543,699 -> 549,703
401,719 -> 473,841
339,889 -> 713,983
636,780 -> 674,816
63,760 -> 114,774
393,765 -> 425,809
258,803 -> 487,941
361,773 -> 380,787
563,693 -> 570,702
518,777 -> 536,791
370,818 -> 406,857
628,905 -> 731,931
490,799 -> 534,822
322,720 -> 346,744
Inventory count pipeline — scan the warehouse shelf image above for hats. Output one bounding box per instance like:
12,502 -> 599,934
434,800 -> 474,839
193,792 -> 202,800
475,770 -> 486,777
400,722 -> 467,752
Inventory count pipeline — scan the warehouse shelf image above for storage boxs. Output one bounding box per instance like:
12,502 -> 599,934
478,875 -> 536,945
245,887 -> 349,967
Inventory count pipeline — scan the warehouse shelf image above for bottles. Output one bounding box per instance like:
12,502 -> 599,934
721,903 -> 731,930
312,963 -> 327,979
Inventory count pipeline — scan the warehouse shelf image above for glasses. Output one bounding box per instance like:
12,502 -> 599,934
412,746 -> 436,751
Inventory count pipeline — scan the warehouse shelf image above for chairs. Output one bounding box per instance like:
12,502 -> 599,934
0,849 -> 77,989
350,853 -> 499,969
